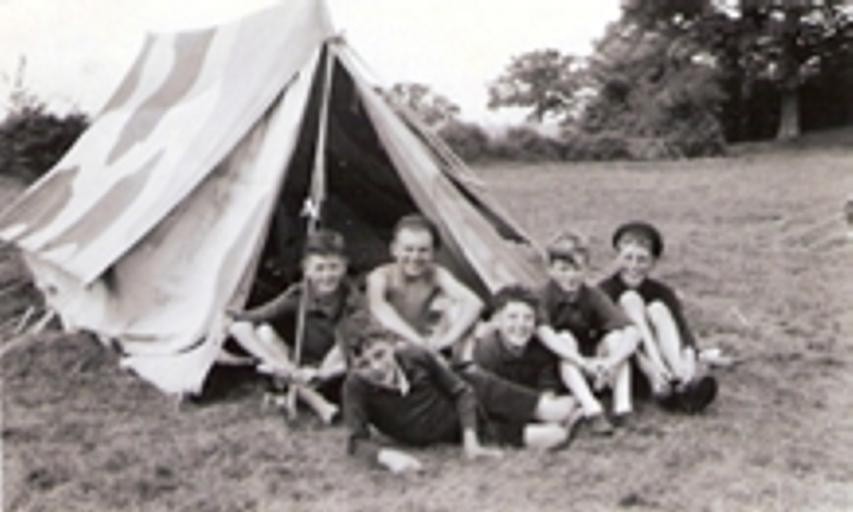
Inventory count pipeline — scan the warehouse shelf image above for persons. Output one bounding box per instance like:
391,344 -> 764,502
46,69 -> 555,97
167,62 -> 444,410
331,305 -> 583,478
456,280 -> 567,399
229,227 -> 364,411
360,212 -> 490,362
530,231 -> 655,442
596,219 -> 718,420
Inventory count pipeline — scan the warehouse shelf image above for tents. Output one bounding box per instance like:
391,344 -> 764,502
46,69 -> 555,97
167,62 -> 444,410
0,1 -> 550,398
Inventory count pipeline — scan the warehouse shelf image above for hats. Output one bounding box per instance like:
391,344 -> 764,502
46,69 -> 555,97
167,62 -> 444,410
614,221 -> 662,258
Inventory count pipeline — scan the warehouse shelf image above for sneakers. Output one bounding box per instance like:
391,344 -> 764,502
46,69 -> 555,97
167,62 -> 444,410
585,414 -> 645,435
262,392 -> 288,415
657,375 -> 718,412
